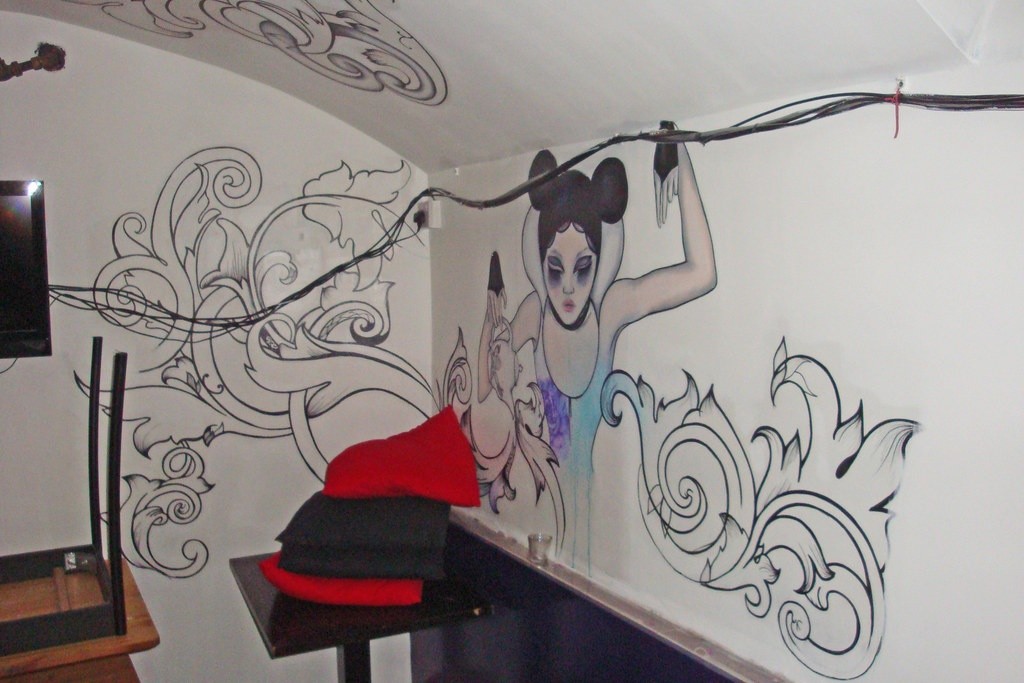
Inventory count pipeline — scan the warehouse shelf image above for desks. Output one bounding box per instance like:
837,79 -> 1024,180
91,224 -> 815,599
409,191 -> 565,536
228,546 -> 497,683
0,558 -> 142,683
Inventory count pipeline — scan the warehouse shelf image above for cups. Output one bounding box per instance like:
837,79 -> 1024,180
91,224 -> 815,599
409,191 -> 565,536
528,533 -> 552,566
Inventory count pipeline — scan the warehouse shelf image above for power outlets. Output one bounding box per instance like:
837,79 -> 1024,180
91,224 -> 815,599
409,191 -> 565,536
417,199 -> 442,230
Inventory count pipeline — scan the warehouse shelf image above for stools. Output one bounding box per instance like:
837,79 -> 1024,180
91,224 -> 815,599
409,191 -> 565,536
0,338 -> 160,677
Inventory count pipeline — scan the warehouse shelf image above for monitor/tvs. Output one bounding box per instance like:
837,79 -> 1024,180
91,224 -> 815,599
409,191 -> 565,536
0,179 -> 52,359
